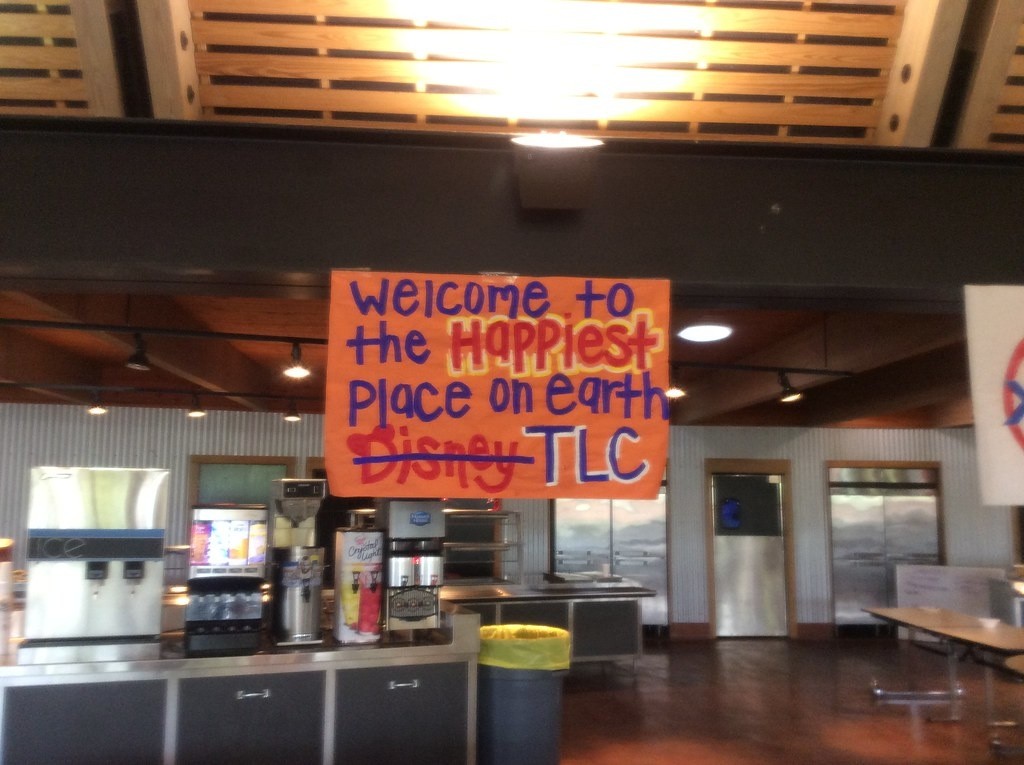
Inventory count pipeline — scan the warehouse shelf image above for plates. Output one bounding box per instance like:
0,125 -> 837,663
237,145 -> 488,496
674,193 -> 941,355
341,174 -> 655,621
919,605 -> 942,611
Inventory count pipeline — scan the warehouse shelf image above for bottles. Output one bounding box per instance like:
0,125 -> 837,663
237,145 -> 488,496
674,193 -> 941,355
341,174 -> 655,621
191,519 -> 267,567
188,591 -> 261,620
0,538 -> 26,666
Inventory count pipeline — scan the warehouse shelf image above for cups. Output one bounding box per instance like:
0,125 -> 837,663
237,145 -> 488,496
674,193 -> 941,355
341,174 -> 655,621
602,563 -> 610,577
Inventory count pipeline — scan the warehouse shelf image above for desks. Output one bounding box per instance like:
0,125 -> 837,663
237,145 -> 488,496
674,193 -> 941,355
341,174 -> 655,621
861,606 -> 999,724
921,625 -> 1024,760
992,654 -> 1024,765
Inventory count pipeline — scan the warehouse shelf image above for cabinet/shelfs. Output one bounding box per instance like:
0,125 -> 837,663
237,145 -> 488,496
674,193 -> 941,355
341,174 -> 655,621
442,508 -> 524,586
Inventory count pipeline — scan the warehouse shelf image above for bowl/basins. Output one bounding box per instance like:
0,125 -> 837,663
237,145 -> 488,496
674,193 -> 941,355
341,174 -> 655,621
979,618 -> 1000,628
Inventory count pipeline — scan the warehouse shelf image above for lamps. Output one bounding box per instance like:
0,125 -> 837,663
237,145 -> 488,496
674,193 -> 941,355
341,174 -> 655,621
88,390 -> 107,414
282,398 -> 301,421
188,392 -> 206,417
126,334 -> 151,371
777,373 -> 802,402
282,342 -> 313,378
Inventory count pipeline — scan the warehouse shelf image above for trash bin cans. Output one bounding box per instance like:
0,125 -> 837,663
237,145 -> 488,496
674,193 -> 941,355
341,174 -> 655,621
477,623 -> 570,765
